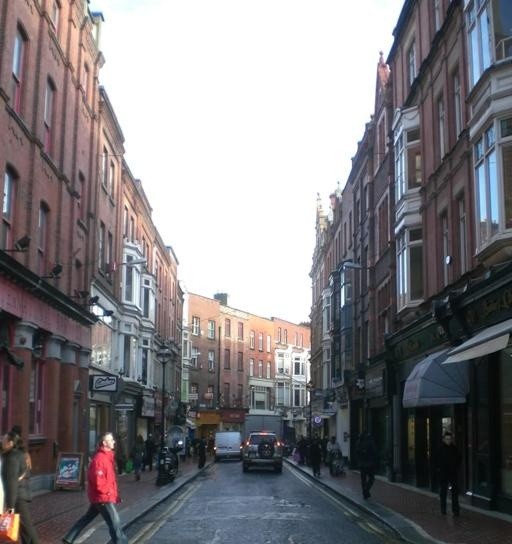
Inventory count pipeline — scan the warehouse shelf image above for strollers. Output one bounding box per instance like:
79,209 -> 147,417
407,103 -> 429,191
331,448 -> 347,476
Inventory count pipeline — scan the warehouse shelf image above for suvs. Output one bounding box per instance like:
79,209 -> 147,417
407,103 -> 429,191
241,430 -> 284,476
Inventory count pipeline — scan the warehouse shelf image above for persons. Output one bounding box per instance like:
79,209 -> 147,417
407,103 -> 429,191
0,431 -> 41,543
434,431 -> 460,516
359,430 -> 376,499
184,436 -> 214,469
7,426 -> 32,478
141,435 -> 156,472
281,433 -> 340,478
130,434 -> 147,481
62,431 -> 130,543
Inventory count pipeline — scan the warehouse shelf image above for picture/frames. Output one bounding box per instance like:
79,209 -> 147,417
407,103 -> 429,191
54,451 -> 85,487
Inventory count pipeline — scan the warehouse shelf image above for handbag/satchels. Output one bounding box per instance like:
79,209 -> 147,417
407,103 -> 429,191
1,513 -> 20,542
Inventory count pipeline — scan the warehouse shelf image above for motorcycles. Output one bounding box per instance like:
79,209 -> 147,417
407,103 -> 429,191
159,445 -> 179,482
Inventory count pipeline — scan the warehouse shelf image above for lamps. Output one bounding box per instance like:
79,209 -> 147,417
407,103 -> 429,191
68,289 -> 90,299
95,311 -> 113,317
80,295 -> 99,306
40,263 -> 64,279
2,236 -> 31,252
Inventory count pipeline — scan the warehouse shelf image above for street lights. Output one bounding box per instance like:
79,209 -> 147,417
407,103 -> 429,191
155,339 -> 171,487
306,380 -> 315,437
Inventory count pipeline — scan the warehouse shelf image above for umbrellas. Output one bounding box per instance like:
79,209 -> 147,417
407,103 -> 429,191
351,443 -> 387,466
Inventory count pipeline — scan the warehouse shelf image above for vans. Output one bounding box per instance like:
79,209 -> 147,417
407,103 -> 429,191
213,431 -> 242,462
